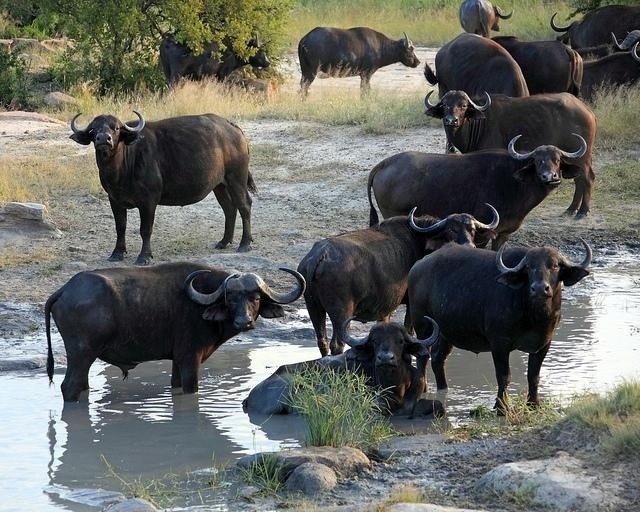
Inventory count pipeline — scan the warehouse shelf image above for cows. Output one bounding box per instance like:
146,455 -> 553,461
368,134 -> 588,251
425,33 -> 529,154
424,0 -> 636,95
298,27 -> 420,104
408,237 -> 592,416
243,316 -> 445,420
68,111 -> 257,266
424,91 -> 596,219
298,202 -> 500,358
45,263 -> 306,402
160,30 -> 270,89
